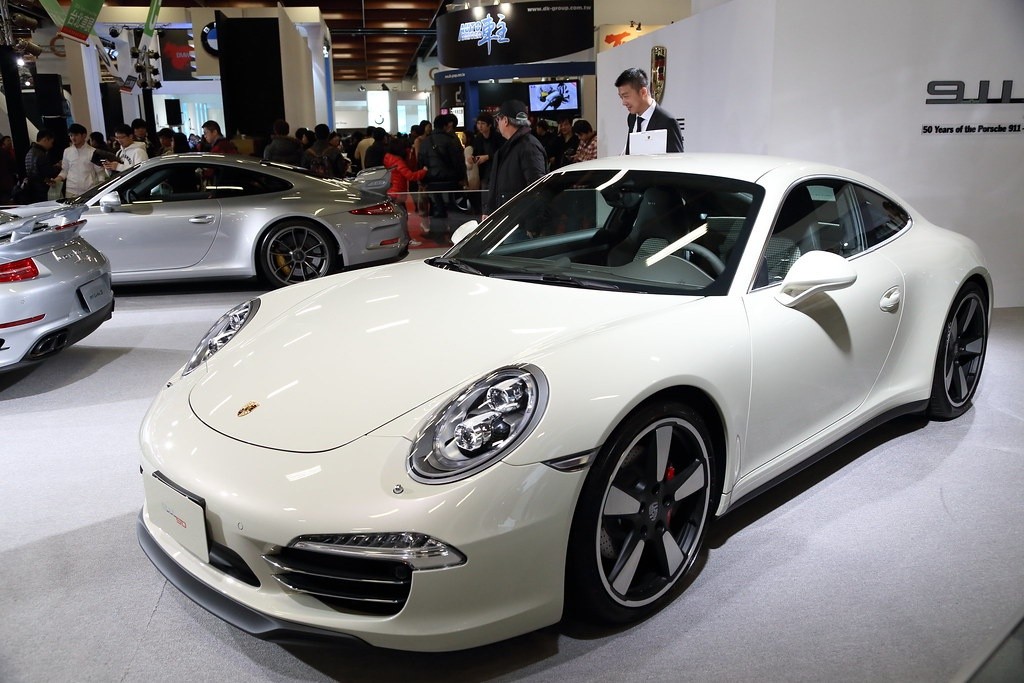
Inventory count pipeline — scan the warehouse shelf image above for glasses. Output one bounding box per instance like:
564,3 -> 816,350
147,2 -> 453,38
115,136 -> 126,140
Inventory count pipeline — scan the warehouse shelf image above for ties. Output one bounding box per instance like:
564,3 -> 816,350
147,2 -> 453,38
636,116 -> 645,132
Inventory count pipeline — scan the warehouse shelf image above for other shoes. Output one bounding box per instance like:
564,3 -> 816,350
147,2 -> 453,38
420,223 -> 429,232
408,240 -> 422,247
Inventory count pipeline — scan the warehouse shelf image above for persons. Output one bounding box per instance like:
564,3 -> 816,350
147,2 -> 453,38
463,114 -> 506,214
347,114 -> 466,244
46,124 -> 105,198
529,114 -> 597,171
0,128 -> 62,204
615,67 -> 684,156
87,119 -> 239,197
481,101 -> 550,244
263,121 -> 350,178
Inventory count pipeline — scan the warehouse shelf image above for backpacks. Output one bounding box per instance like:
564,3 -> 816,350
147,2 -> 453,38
307,146 -> 334,177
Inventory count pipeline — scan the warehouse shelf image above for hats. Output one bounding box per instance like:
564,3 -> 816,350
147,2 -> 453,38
490,99 -> 529,120
328,130 -> 341,142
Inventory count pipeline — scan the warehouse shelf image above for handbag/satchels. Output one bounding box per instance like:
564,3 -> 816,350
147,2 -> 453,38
47,178 -> 64,201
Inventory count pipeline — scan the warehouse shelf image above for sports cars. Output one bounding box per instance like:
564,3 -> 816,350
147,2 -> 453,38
136,153 -> 998,652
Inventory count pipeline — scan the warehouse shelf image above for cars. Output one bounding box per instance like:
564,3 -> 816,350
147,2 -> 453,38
1,209 -> 117,371
7,152 -> 409,291
